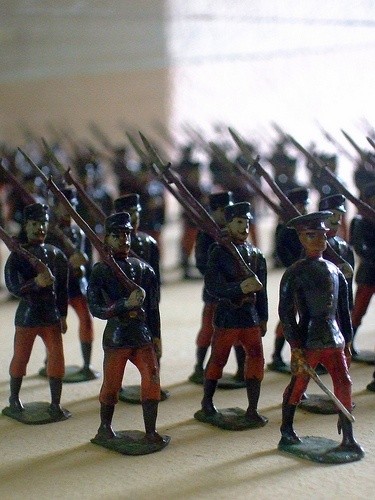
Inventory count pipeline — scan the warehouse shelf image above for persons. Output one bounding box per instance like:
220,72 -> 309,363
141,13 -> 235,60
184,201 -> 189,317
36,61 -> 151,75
0,143 -> 375,399
86,212 -> 166,446
278,211 -> 369,456
201,198 -> 268,426
4,203 -> 69,418
